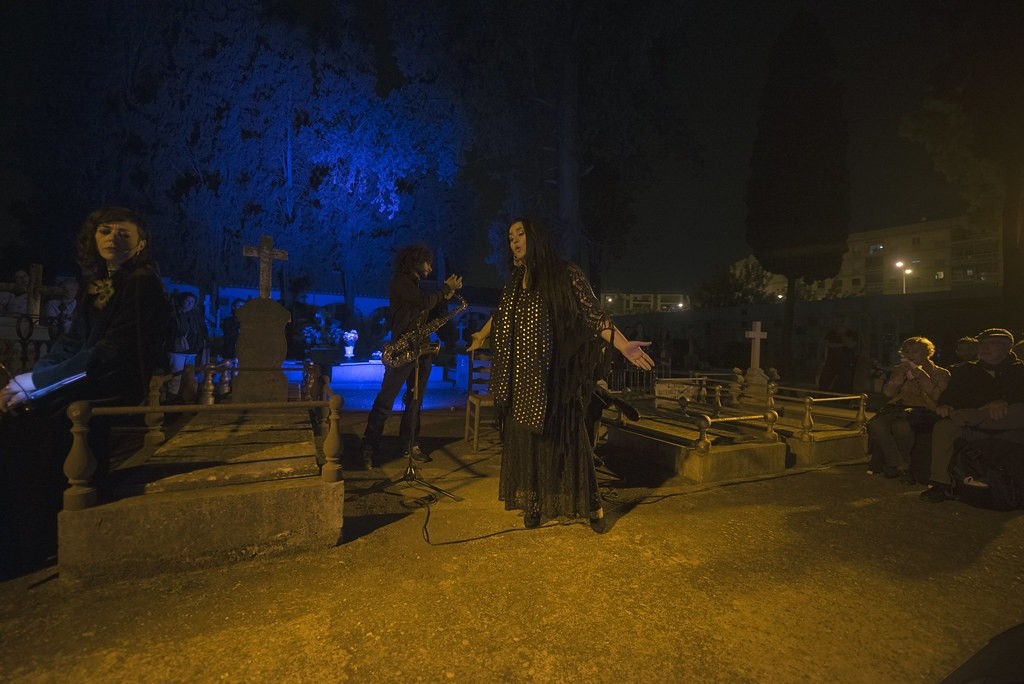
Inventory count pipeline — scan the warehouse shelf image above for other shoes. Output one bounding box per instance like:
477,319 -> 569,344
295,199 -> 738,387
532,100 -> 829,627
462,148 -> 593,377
921,486 -> 960,502
524,511 -> 540,528
589,511 -> 605,533
884,467 -> 897,478
898,469 -> 915,484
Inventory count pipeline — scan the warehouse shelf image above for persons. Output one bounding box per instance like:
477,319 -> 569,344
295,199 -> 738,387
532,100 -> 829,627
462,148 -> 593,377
1,203 -> 174,564
168,293 -> 205,401
868,327 -> 1024,509
1,270 -> 32,314
222,299 -> 247,371
617,321 -> 677,372
43,276 -> 89,333
820,314 -> 860,405
359,243 -> 463,471
466,218 -> 655,534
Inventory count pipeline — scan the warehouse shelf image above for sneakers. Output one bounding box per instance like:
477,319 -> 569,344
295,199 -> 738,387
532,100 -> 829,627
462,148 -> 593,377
401,443 -> 430,463
359,443 -> 377,469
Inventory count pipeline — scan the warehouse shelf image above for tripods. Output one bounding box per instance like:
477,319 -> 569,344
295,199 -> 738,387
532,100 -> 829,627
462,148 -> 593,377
358,320 -> 462,504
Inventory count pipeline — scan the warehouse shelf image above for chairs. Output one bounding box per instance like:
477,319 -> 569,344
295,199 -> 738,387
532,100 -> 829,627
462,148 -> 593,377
464,348 -> 498,453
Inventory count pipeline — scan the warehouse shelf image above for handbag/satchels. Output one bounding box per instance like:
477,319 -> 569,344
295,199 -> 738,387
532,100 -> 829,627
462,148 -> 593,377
950,437 -> 1024,511
907,410 -> 941,433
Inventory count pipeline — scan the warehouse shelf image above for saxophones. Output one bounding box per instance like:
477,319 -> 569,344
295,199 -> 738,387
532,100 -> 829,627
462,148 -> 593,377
382,290 -> 468,368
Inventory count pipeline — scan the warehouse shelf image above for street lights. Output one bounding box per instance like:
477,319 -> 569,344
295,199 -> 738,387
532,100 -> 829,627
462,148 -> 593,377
895,260 -> 913,293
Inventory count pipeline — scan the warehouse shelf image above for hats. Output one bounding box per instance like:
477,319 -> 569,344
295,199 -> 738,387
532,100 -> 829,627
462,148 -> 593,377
975,329 -> 1015,346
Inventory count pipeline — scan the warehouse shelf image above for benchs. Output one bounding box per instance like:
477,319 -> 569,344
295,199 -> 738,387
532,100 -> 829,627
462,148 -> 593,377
879,364 -> 1024,509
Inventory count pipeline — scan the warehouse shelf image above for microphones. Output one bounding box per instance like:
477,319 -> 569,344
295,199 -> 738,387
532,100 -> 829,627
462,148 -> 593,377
409,310 -> 428,325
